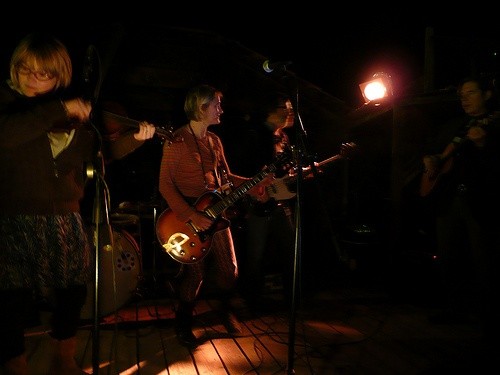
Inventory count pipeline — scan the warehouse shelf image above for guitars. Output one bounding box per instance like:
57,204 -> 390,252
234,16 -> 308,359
257,141 -> 359,204
154,144 -> 296,265
418,126 -> 483,196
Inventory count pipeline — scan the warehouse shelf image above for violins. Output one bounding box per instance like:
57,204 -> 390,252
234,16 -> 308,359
92,102 -> 185,145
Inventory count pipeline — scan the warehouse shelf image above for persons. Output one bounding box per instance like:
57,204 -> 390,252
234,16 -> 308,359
158,84 -> 278,348
421,74 -> 500,325
234,92 -> 324,319
0,34 -> 156,375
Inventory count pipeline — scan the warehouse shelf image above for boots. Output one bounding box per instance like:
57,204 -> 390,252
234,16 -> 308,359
5,352 -> 31,375
48,332 -> 90,375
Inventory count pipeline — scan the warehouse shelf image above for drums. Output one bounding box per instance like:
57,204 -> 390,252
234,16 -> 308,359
79,228 -> 142,320
111,214 -> 140,242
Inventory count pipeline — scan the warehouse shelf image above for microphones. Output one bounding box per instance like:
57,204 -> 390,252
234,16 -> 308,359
83,45 -> 93,81
262,60 -> 292,73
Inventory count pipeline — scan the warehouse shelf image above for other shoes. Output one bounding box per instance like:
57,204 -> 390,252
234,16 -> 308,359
220,312 -> 241,334
174,325 -> 197,347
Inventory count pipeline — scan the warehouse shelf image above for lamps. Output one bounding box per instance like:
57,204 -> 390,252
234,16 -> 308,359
359,72 -> 394,106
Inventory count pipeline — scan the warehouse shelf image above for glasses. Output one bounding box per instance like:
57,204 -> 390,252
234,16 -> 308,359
14,61 -> 57,82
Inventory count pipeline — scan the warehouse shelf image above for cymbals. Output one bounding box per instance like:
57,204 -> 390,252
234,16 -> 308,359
119,203 -> 159,208
146,200 -> 160,203
